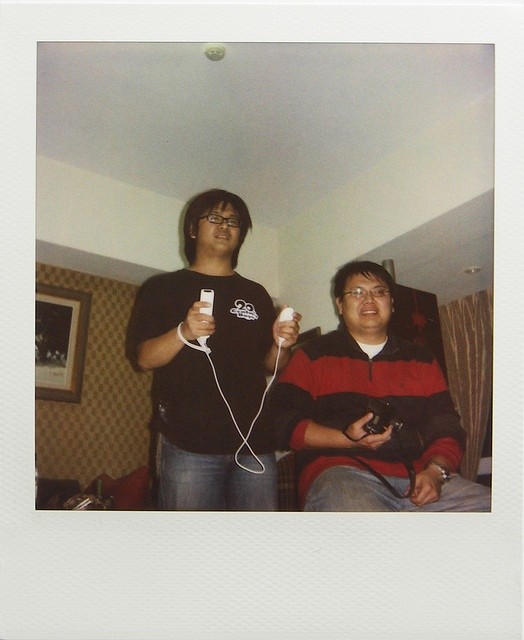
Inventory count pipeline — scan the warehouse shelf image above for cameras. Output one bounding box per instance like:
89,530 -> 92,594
365,398 -> 404,437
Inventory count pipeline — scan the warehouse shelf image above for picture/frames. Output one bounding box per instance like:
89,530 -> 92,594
35,283 -> 91,404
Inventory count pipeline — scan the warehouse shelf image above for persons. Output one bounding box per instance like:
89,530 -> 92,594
263,259 -> 492,513
125,189 -> 303,511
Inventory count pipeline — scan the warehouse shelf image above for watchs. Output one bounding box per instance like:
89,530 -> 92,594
426,459 -> 451,486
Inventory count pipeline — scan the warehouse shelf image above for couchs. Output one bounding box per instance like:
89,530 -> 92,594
39,467 -> 154,510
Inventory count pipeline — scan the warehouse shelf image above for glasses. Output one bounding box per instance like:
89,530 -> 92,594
198,214 -> 242,227
339,285 -> 392,301
